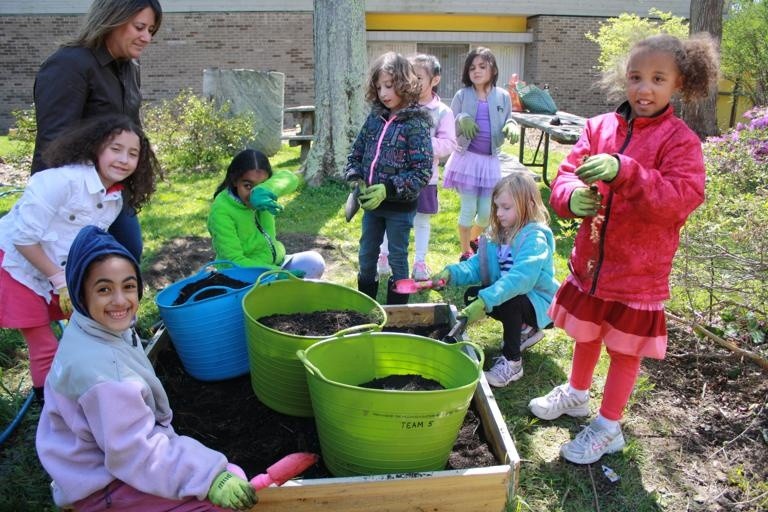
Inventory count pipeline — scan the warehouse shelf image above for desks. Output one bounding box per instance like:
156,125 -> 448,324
509,110 -> 589,189
284,106 -> 315,162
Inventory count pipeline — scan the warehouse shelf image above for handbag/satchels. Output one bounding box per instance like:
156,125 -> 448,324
508,74 -> 557,114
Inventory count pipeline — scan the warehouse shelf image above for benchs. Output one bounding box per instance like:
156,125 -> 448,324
280,135 -> 318,165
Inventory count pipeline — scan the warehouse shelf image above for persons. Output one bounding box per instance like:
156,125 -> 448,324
2,110 -> 165,409
30,0 -> 162,264
437,172 -> 559,388
528,31 -> 723,467
442,47 -> 519,260
34,224 -> 260,511
207,148 -> 327,284
346,50 -> 432,308
372,54 -> 455,280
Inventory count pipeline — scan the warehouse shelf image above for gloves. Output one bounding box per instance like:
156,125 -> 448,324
47,270 -> 73,315
459,117 -> 480,141
349,178 -> 386,211
209,467 -> 259,510
575,153 -> 620,185
568,184 -> 604,217
279,268 -> 307,279
456,297 -> 485,328
252,192 -> 284,214
430,269 -> 450,291
502,122 -> 519,145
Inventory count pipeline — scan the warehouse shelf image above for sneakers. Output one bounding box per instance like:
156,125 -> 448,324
529,383 -> 590,421
412,260 -> 429,281
559,416 -> 625,464
470,238 -> 480,254
486,355 -> 523,387
377,253 -> 391,275
459,250 -> 474,261
500,324 -> 545,353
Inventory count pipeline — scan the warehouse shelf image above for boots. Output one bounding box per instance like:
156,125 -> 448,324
386,276 -> 410,305
358,271 -> 379,301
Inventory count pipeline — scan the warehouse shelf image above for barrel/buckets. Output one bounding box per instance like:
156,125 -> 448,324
154,261 -> 279,381
295,332 -> 485,477
242,270 -> 388,416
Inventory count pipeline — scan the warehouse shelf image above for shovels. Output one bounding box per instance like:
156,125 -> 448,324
344,184 -> 360,222
